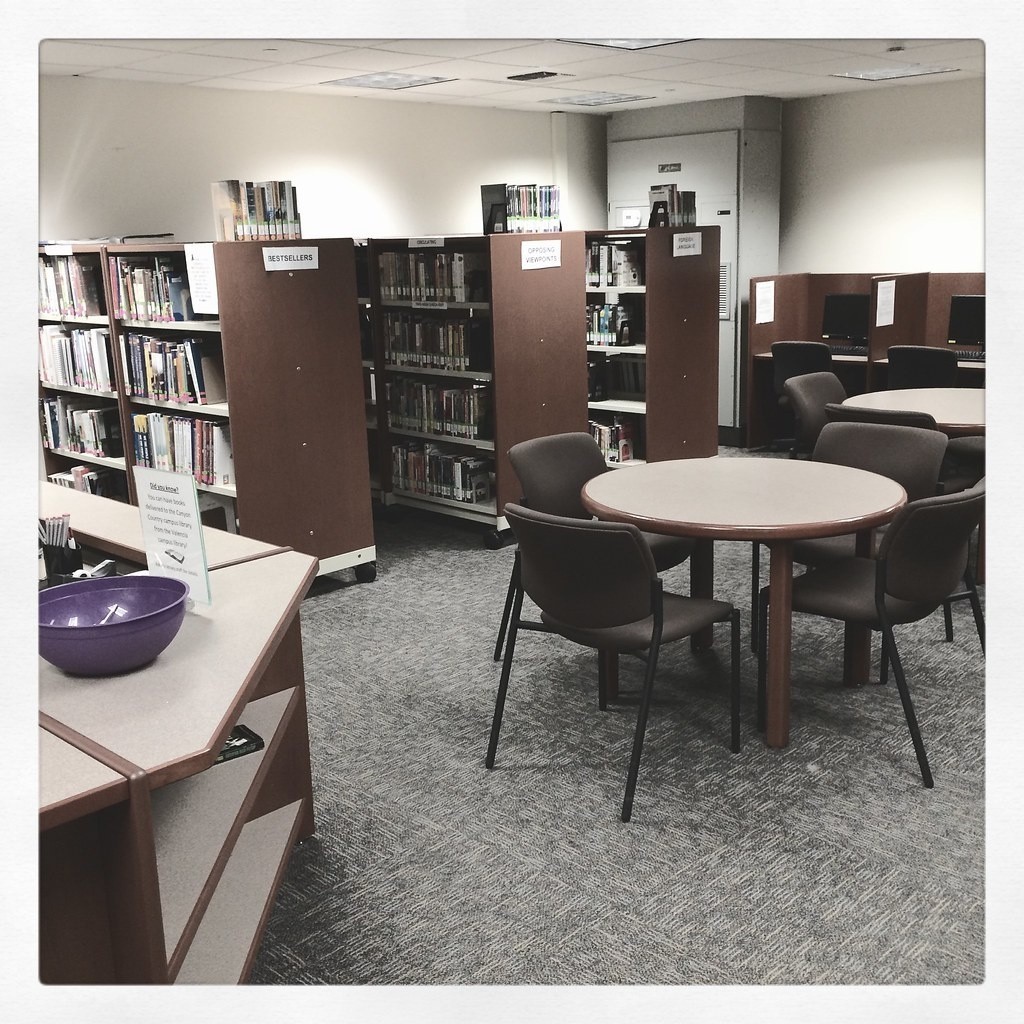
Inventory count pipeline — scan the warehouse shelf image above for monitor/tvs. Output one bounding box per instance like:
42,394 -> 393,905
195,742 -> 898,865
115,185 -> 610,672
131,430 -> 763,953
948,295 -> 985,351
822,294 -> 871,346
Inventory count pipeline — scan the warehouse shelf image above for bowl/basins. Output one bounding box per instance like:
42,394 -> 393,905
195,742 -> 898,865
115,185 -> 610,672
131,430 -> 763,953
39,576 -> 190,676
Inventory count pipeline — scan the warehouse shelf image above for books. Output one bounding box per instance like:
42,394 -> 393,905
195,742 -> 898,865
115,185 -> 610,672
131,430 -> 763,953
648,184 -> 696,227
38,237 -> 109,246
480,184 -> 561,235
358,251 -> 490,503
211,180 -> 302,241
113,232 -> 175,245
39,255 -> 239,533
585,239 -> 644,462
213,724 -> 265,765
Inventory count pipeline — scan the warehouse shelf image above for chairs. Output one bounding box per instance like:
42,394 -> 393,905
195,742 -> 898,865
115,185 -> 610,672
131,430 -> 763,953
492,431 -> 698,674
477,503 -> 740,824
757,474 -> 986,789
752,341 -> 985,656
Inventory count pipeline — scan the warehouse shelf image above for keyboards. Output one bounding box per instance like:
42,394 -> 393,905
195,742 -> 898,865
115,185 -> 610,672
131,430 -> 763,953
955,350 -> 986,362
830,345 -> 869,356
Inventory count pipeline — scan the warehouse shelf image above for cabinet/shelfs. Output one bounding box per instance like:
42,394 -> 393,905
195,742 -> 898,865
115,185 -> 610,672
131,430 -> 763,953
38,219 -> 722,579
38,477 -> 318,985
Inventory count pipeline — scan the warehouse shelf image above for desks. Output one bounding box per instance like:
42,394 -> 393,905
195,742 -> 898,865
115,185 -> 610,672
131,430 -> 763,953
582,456 -> 907,758
872,357 -> 986,374
841,388 -> 985,443
753,350 -> 868,368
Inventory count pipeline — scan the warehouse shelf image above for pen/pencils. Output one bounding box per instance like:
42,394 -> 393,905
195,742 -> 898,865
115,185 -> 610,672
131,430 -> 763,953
38,512 -> 76,549
